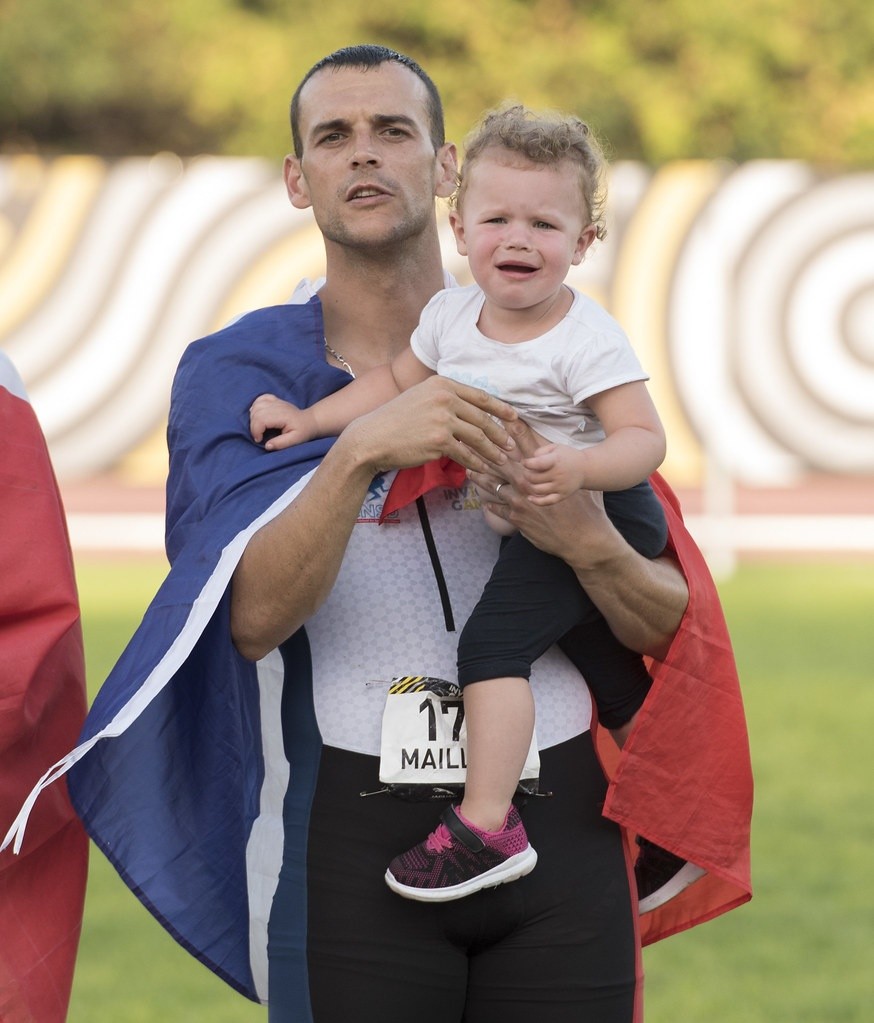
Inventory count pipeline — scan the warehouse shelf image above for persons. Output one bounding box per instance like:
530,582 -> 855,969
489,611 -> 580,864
167,44 -> 690,1023
250,106 -> 711,915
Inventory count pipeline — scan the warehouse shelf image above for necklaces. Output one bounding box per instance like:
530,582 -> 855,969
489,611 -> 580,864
324,337 -> 355,378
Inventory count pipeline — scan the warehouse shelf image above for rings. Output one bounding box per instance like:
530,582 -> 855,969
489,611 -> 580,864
496,483 -> 510,501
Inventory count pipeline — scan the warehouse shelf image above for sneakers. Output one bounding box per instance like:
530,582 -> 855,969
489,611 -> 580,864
384,803 -> 538,901
633,837 -> 705,915
